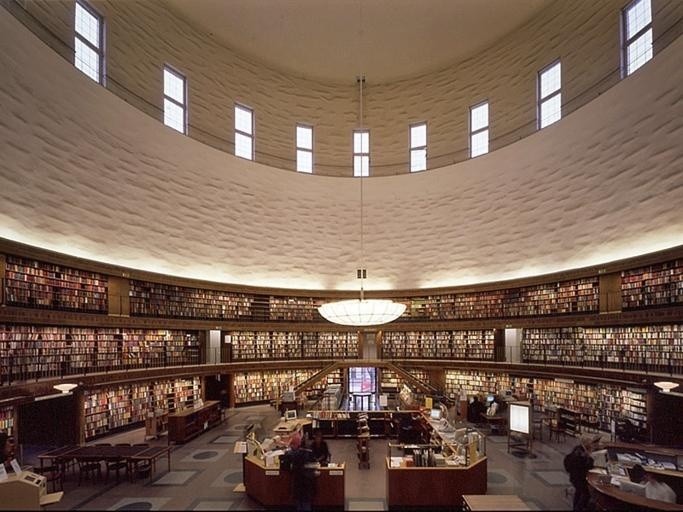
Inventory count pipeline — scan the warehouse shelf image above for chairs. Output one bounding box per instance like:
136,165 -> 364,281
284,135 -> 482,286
35,439 -> 172,492
468,404 -> 566,443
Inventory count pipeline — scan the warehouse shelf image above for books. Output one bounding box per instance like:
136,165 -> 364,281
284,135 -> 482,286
603,452 -> 675,486
412,448 -> 439,467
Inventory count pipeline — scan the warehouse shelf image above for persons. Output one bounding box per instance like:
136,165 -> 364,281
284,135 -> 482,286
0,432 -> 20,473
644,473 -> 677,503
564,441 -> 595,512
281,437 -> 316,512
310,434 -> 328,465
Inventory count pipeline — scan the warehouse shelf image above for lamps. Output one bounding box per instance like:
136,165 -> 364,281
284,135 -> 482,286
316,74 -> 407,326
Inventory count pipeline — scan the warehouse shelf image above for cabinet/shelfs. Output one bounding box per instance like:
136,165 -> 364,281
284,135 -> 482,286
362,282 -> 498,408
236,285 -> 362,407
0,237 -> 234,470
497,245 -> 683,433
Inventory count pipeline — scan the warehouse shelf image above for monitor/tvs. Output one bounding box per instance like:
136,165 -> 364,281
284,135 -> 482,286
487,395 -> 495,402
284,409 -> 298,420
500,389 -> 512,398
590,448 -> 608,468
453,427 -> 467,442
430,408 -> 442,419
618,479 -> 646,496
